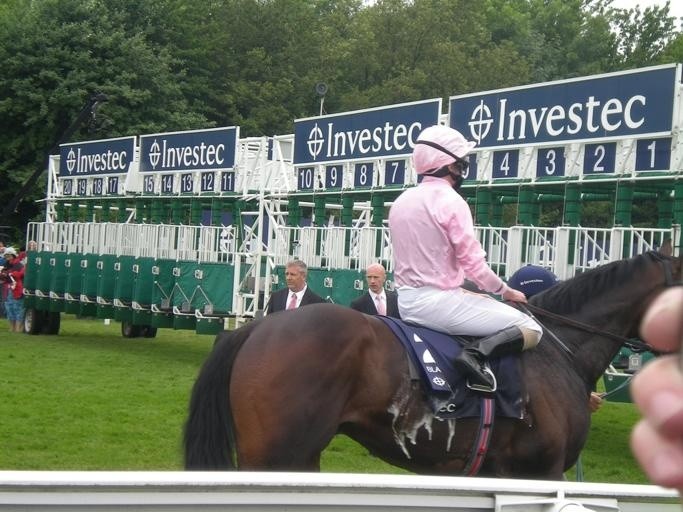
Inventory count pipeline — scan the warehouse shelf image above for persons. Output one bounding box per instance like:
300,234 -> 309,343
265,258 -> 327,315
0,246 -> 28,332
628,286 -> 683,491
0,236 -> 10,319
589,391 -> 607,412
350,262 -> 402,320
389,123 -> 545,400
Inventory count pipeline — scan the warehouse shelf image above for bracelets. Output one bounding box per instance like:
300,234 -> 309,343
496,282 -> 504,293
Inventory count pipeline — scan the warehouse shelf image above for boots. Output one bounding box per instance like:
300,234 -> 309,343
451,323 -> 525,401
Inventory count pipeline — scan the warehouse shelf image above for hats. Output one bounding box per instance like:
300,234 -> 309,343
410,123 -> 478,176
0,247 -> 18,258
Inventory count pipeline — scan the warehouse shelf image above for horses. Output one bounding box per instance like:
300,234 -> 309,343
181,238 -> 683,481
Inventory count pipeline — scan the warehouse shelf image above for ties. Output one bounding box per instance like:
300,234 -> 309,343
374,294 -> 386,316
287,293 -> 297,311
